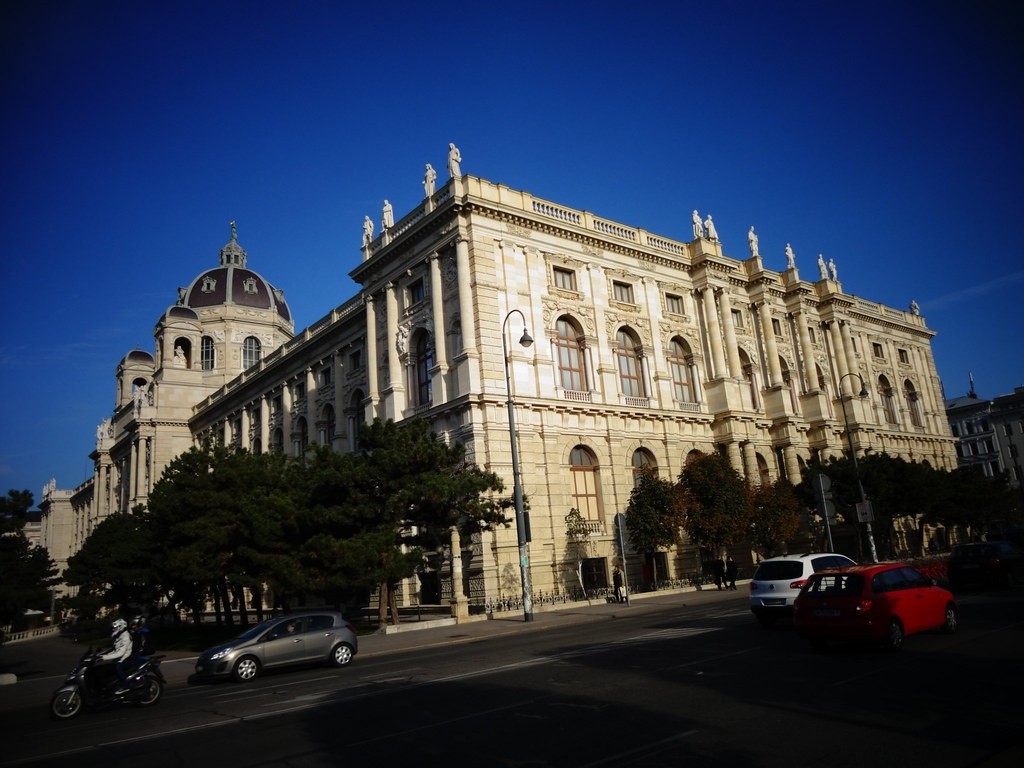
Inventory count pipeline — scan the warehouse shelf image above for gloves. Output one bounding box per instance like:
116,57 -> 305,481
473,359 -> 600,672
94,654 -> 103,661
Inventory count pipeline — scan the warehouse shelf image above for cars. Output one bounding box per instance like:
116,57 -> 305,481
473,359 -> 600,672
749,553 -> 862,628
196,609 -> 359,684
948,542 -> 1024,596
792,559 -> 960,651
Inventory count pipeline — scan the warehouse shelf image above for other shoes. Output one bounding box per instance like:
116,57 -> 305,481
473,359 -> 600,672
114,686 -> 130,695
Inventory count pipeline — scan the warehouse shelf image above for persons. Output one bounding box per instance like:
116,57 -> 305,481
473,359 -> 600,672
91,614 -> 151,694
692,210 -> 704,239
726,556 -> 738,592
422,163 -> 436,199
747,226 -> 760,257
785,243 -> 796,269
713,556 -> 730,591
133,386 -> 154,409
42,478 -> 55,497
96,420 -> 114,440
909,300 -> 921,316
816,253 -> 828,280
380,200 -> 394,233
613,565 -> 629,603
704,214 -> 721,243
362,216 -> 374,248
827,259 -> 838,281
286,622 -> 296,633
447,144 -> 462,178
176,346 -> 185,361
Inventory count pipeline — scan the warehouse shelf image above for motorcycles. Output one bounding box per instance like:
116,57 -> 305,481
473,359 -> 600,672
51,644 -> 166,720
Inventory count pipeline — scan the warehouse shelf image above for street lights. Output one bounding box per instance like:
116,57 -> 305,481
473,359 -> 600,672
502,309 -> 535,624
838,373 -> 880,563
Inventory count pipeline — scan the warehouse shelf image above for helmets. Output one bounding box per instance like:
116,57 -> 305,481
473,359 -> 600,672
129,615 -> 147,632
111,619 -> 128,637
